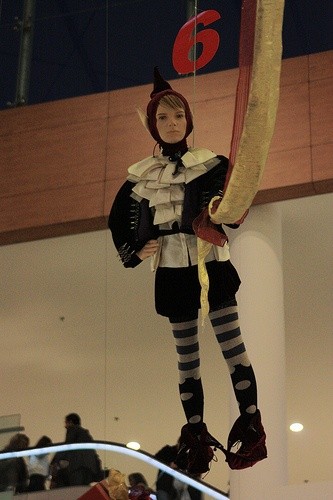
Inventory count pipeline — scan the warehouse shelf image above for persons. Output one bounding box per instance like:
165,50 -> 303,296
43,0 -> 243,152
107,74 -> 267,476
58,411 -> 93,487
0,433 -> 29,499
26,434 -> 53,493
75,467 -> 130,500
126,472 -> 152,500
151,445 -> 203,500
